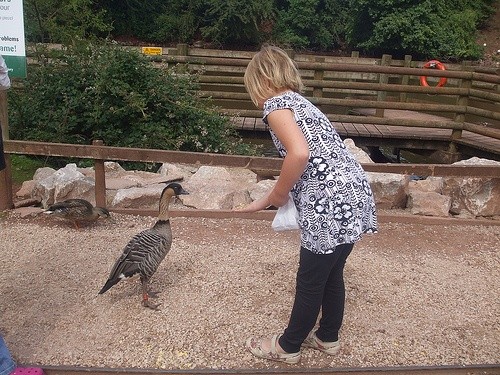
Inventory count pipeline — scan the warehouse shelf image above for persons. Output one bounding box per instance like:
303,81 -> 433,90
0,336 -> 44,375
231,44 -> 378,366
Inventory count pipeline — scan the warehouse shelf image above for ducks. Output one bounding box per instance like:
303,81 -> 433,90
97,182 -> 189,311
40,198 -> 116,230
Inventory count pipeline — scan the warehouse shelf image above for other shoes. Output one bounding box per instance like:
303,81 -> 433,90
10,365 -> 46,375
301,331 -> 341,355
247,333 -> 301,364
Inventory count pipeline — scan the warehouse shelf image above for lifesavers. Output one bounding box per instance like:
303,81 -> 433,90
419,60 -> 447,88
